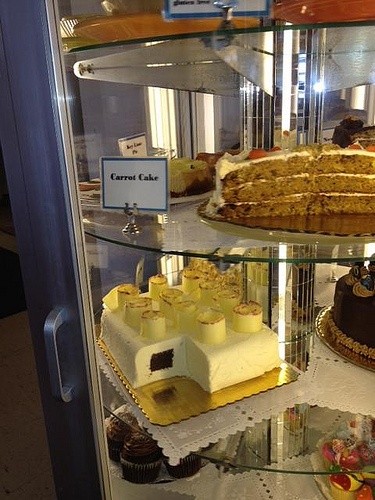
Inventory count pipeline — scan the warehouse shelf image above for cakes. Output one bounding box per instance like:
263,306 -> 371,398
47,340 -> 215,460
99,265 -> 282,395
322,254 -> 375,368
169,116 -> 375,222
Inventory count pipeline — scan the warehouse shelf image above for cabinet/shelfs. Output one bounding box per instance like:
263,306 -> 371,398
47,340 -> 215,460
43,0 -> 375,500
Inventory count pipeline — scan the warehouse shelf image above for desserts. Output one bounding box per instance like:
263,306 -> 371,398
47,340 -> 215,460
323,418 -> 375,500
106,410 -> 201,483
